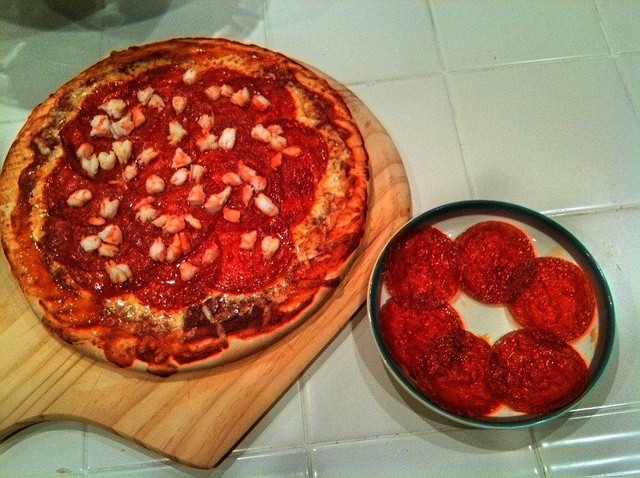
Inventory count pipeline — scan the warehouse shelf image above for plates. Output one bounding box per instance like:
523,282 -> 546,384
367,199 -> 616,430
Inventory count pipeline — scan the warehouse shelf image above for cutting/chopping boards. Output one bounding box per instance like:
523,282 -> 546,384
1,56 -> 413,470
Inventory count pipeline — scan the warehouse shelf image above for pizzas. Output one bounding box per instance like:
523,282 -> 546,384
1,38 -> 371,375
